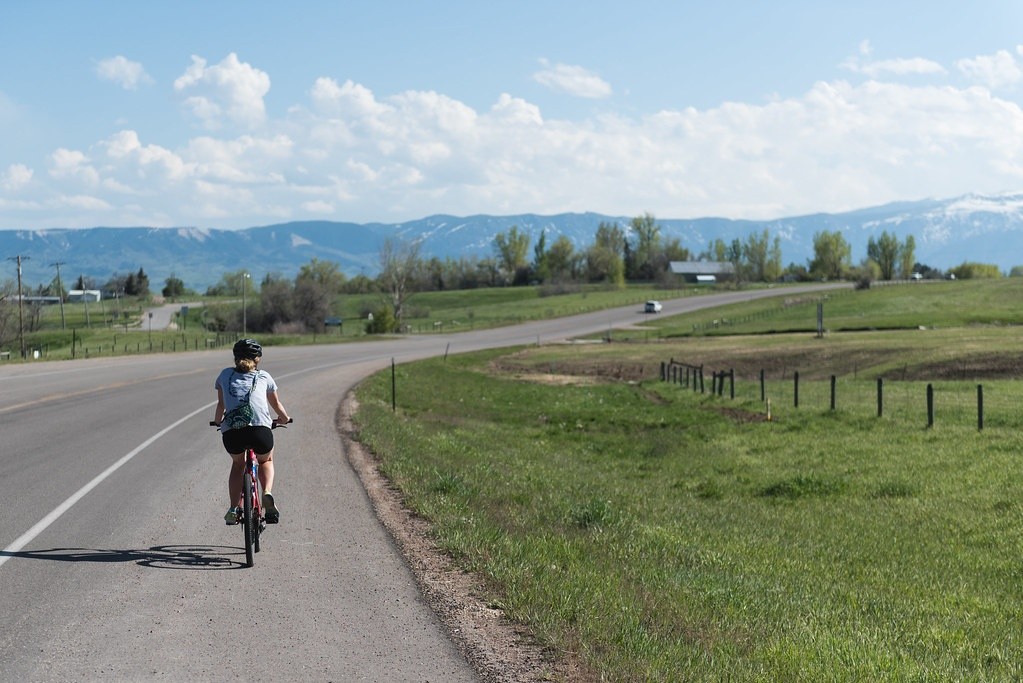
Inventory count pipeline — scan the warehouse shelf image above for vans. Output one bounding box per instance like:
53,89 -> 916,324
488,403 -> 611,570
645,301 -> 662,313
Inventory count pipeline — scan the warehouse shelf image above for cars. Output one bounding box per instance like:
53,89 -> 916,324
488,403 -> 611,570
911,271 -> 923,279
325,316 -> 343,327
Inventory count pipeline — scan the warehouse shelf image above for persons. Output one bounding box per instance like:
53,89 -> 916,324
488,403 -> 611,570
214,338 -> 290,524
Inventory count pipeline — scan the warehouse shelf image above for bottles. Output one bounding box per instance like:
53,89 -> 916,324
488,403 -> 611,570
252,461 -> 257,478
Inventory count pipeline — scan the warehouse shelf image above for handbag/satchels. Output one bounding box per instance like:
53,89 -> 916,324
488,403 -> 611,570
223,371 -> 259,429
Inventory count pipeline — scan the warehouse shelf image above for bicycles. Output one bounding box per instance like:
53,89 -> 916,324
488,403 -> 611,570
208,415 -> 295,567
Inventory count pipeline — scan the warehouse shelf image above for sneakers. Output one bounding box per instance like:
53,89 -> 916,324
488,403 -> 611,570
262,492 -> 280,520
225,506 -> 237,525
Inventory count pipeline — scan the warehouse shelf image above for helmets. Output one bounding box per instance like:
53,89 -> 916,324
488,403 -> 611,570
233,338 -> 262,359
